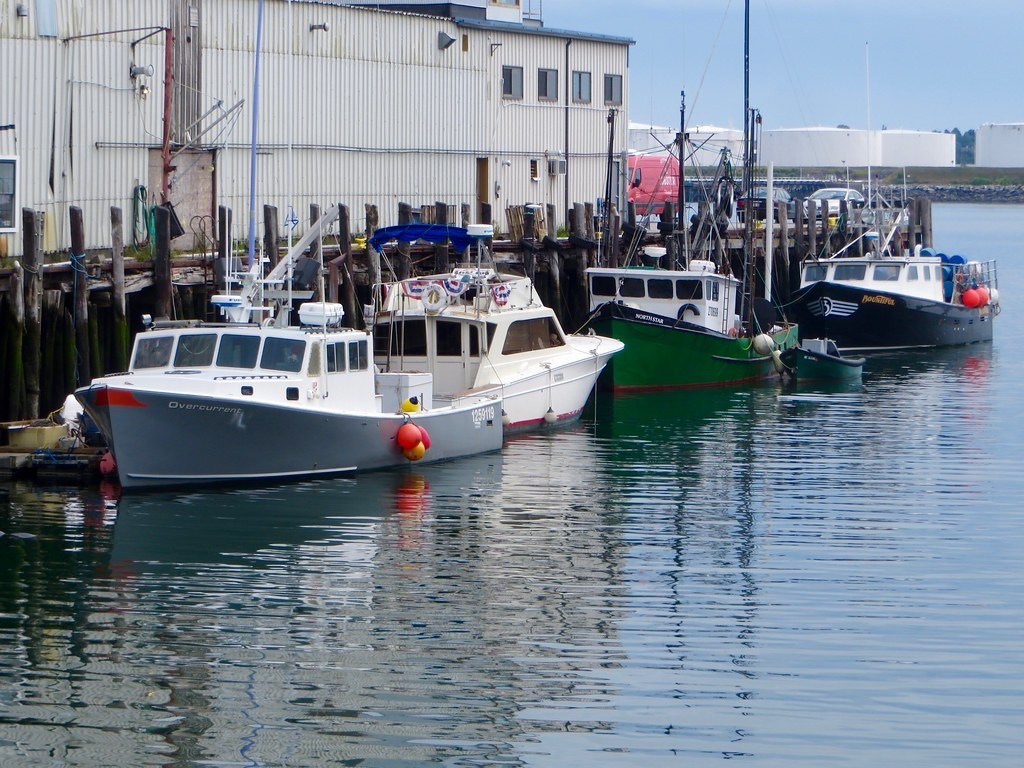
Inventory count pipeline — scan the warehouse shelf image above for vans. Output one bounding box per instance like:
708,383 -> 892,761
627,154 -> 680,223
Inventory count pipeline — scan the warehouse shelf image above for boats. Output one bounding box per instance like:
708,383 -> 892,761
776,336 -> 868,384
569,87 -> 803,396
782,160 -> 1004,359
77,135 -> 504,500
573,370 -> 782,535
358,222 -> 627,441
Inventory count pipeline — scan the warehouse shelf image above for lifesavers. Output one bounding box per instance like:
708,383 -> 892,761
691,214 -> 711,235
656,222 -> 678,230
678,304 -> 701,324
622,221 -> 647,245
519,237 -> 539,252
421,284 -> 447,311
543,235 -> 563,251
569,235 -> 595,249
707,214 -> 729,229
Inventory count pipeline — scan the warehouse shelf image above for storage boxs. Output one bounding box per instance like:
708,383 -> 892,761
8,424 -> 70,449
375,372 -> 433,412
802,339 -> 827,355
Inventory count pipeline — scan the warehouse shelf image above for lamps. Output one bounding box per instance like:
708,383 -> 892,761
141,314 -> 155,332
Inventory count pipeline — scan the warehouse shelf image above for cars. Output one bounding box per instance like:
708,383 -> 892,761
738,186 -> 796,222
802,187 -> 865,225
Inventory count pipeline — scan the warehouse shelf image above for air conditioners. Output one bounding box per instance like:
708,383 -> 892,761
550,159 -> 567,176
